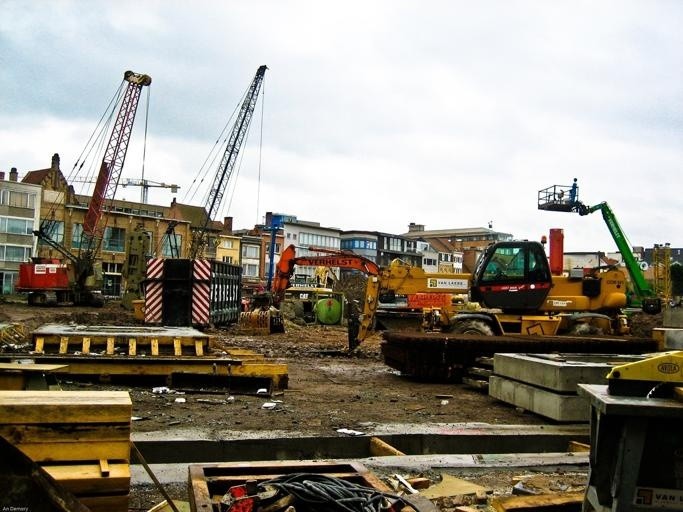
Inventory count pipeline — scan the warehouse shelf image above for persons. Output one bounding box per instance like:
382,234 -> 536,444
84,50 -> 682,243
570,178 -> 577,203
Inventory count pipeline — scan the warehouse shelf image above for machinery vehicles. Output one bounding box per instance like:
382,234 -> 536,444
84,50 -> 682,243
16,70 -> 152,308
